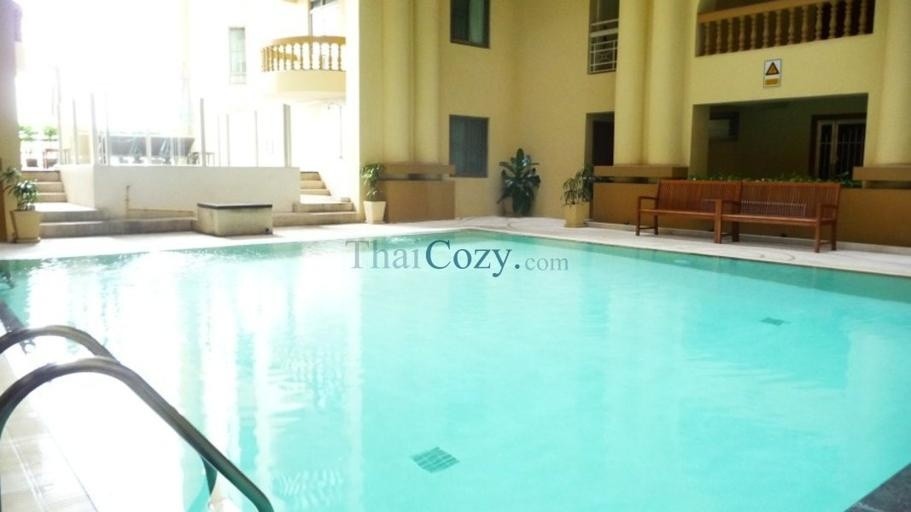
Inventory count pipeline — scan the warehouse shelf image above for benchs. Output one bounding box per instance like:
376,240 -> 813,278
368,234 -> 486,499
635,178 -> 742,253
717,181 -> 841,253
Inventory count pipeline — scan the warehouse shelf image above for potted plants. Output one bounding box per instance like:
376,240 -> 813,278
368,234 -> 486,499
497,148 -> 540,217
362,163 -> 386,223
561,165 -> 593,227
0,167 -> 42,243
